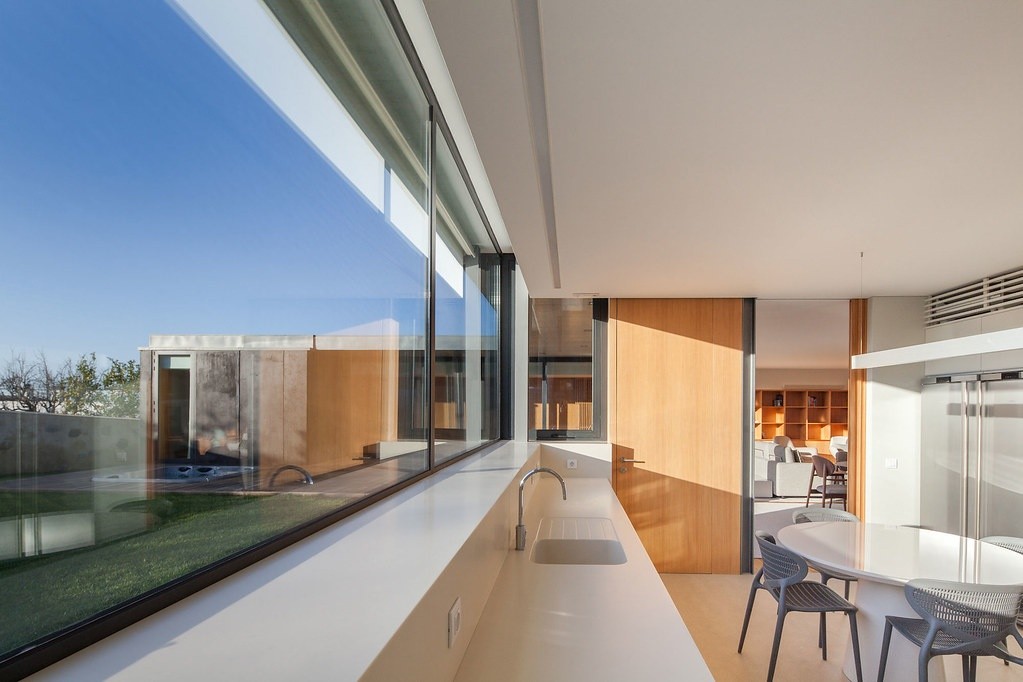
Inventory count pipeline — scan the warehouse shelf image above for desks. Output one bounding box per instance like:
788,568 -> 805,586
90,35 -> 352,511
834,461 -> 850,484
778,522 -> 1023,682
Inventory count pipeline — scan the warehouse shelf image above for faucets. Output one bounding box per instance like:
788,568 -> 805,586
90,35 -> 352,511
515,466 -> 567,551
268,463 -> 314,492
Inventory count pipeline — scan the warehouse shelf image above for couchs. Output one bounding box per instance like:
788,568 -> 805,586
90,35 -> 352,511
755,441 -> 831,498
830,436 -> 848,458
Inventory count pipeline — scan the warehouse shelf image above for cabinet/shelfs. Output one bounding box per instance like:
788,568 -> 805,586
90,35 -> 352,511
755,389 -> 849,454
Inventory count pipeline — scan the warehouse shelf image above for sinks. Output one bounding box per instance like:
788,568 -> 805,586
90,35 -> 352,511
529,538 -> 629,566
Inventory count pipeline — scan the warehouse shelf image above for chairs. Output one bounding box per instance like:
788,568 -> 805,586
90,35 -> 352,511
806,455 -> 848,511
876,578 -> 1023,682
792,508 -> 862,615
738,530 -> 863,682
969,535 -> 1023,682
833,452 -> 847,484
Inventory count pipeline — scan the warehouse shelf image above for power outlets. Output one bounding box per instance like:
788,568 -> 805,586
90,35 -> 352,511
567,459 -> 577,468
448,597 -> 462,649
